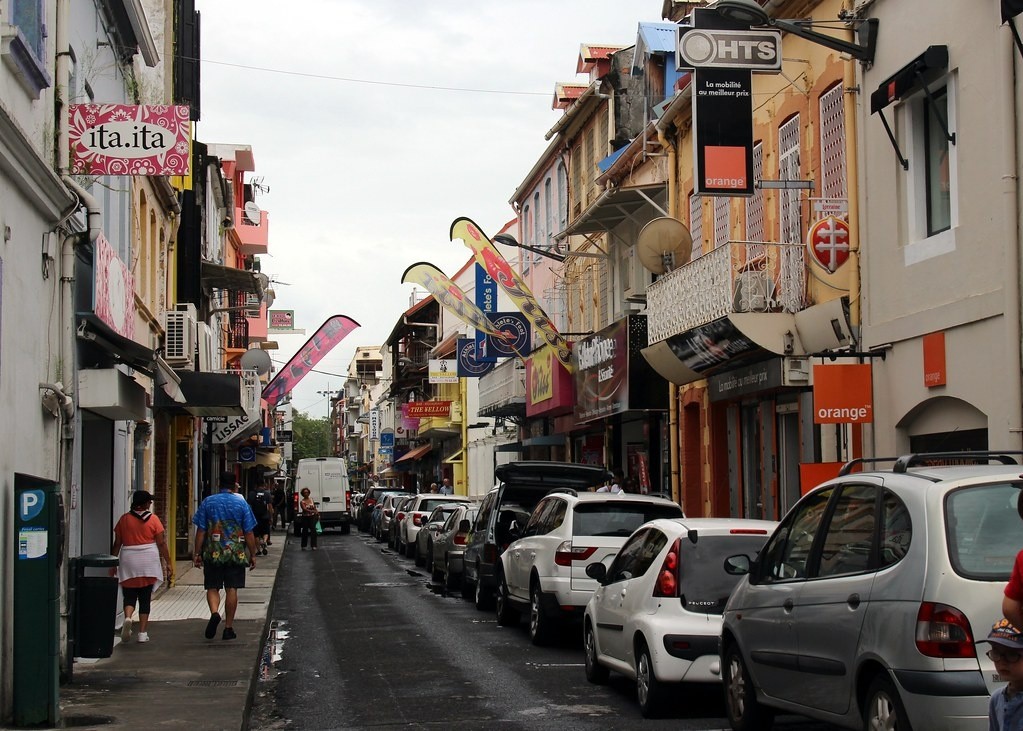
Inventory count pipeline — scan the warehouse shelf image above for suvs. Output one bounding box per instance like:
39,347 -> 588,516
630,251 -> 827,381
718,451 -> 1022,730
493,486 -> 686,647
464,461 -> 615,611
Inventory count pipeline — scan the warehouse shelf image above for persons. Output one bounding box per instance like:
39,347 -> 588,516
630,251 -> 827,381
108,490 -> 173,642
430,483 -> 439,494
193,472 -> 257,640
1001,489 -> 1023,632
440,479 -> 453,494
974,619 -> 1023,731
249,482 -> 272,556
234,482 -> 250,562
271,483 -> 285,529
300,488 -> 319,551
587,470 -> 628,493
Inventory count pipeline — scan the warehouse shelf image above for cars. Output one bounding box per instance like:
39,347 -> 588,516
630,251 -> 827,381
351,486 -> 482,585
582,517 -> 780,716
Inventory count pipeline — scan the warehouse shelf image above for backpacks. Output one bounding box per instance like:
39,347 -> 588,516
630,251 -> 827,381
251,489 -> 267,516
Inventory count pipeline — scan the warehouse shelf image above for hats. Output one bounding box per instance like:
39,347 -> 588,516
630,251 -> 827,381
133,491 -> 156,502
219,471 -> 236,483
974,618 -> 1023,648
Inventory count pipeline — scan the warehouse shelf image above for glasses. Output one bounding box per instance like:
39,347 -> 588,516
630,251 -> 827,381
986,649 -> 1023,663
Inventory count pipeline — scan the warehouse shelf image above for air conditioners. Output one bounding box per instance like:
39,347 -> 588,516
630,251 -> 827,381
164,310 -> 195,366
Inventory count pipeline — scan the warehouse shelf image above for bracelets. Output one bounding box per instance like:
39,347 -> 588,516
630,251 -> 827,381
195,554 -> 200,556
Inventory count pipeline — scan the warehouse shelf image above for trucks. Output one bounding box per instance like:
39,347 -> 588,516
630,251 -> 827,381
293,456 -> 352,535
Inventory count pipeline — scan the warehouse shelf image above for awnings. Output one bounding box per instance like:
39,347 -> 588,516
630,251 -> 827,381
242,451 -> 283,469
81,319 -> 185,402
396,444 -> 432,463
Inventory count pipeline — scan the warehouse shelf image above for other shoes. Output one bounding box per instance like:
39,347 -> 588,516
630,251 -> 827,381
223,627 -> 237,640
137,632 -> 149,643
205,612 -> 221,639
312,547 -> 317,550
256,551 -> 261,556
271,525 -> 275,531
262,542 -> 268,555
267,540 -> 272,545
302,547 -> 307,550
121,617 -> 131,642
281,528 -> 285,531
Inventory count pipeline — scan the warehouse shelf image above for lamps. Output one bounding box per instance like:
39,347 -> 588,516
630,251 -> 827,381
493,233 -> 570,263
715,0 -> 879,71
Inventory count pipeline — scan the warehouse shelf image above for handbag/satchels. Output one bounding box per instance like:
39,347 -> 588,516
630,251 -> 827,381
315,519 -> 323,535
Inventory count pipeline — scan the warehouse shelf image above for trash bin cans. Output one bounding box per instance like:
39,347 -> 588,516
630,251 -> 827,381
72,553 -> 118,658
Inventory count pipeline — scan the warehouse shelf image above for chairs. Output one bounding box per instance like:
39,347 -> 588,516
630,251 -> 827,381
496,511 -> 518,544
